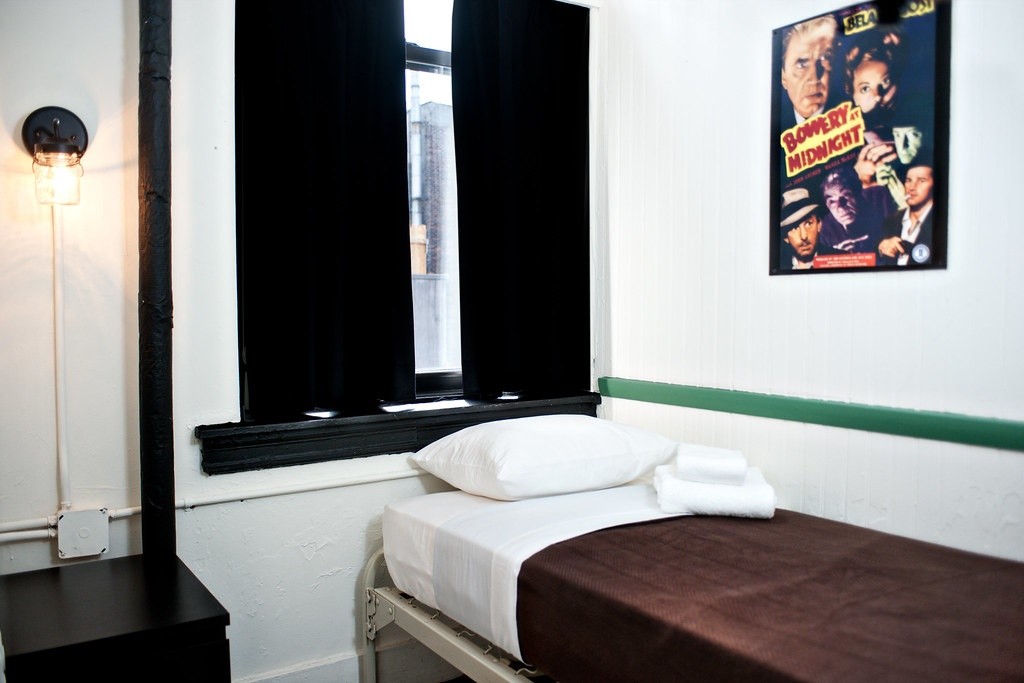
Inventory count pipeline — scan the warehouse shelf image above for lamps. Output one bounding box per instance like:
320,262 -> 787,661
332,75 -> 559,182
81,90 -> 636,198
21,106 -> 89,205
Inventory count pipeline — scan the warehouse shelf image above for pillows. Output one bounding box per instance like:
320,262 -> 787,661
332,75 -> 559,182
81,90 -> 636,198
408,413 -> 676,501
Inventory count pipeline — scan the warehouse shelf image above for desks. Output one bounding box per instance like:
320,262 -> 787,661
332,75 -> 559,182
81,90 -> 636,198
0,551 -> 233,683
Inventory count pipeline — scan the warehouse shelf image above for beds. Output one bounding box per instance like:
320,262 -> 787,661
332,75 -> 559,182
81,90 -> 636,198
363,412 -> 1024,683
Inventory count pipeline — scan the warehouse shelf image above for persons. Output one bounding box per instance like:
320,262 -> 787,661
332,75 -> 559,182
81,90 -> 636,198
778,187 -> 853,270
877,153 -> 946,269
781,12 -> 878,218
878,87 -> 945,215
815,167 -> 892,256
843,42 -> 935,215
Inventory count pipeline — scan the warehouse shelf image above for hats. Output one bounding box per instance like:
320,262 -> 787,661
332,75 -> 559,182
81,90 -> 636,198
780,188 -> 820,226
